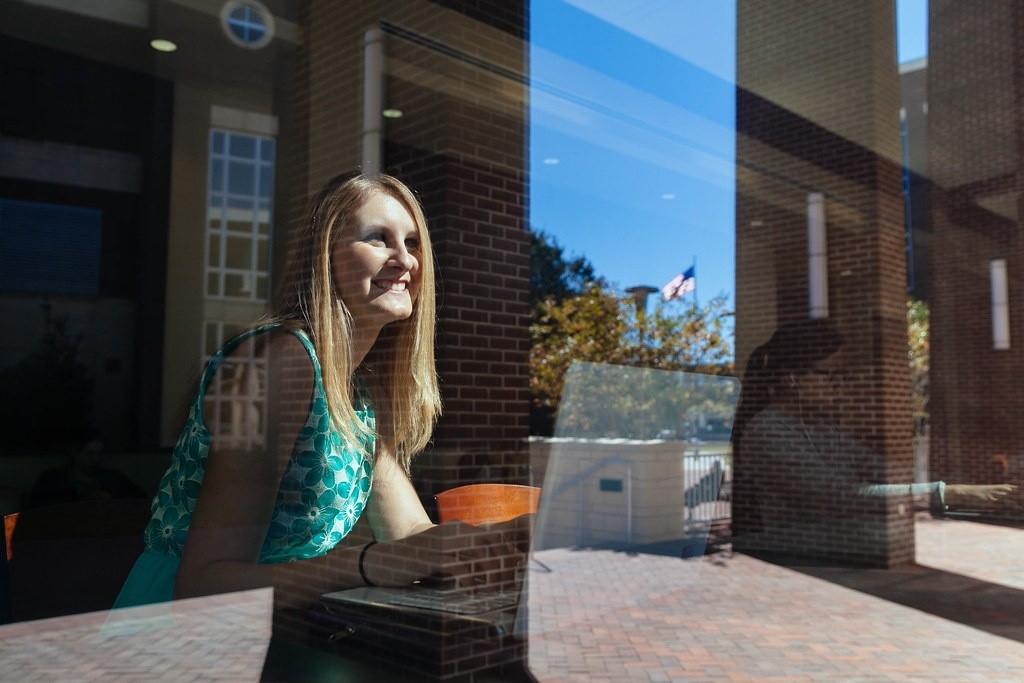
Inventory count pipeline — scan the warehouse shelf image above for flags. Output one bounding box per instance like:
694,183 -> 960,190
662,266 -> 696,303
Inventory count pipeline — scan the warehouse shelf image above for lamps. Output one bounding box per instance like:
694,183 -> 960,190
543,129 -> 560,164
659,171 -> 675,201
146,0 -> 179,52
989,260 -> 1010,351
382,76 -> 403,118
806,193 -> 829,318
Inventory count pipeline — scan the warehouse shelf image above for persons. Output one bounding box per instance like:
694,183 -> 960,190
13,429 -> 139,608
108,174 -> 535,638
731,314 -> 1024,537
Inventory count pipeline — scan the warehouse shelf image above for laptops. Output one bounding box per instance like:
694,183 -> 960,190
320,361 -> 742,642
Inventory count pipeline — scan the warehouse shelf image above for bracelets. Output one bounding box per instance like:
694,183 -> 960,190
359,540 -> 379,588
